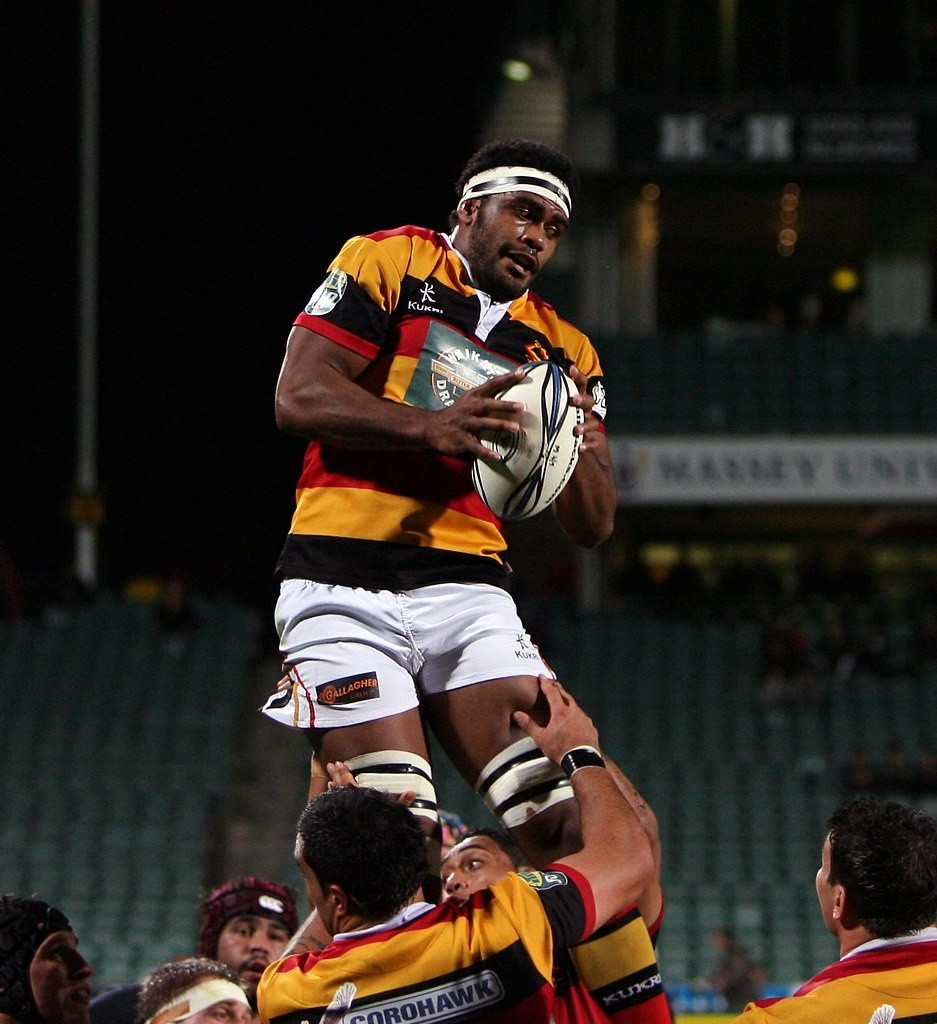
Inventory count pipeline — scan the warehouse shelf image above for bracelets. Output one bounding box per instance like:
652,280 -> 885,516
560,746 -> 606,778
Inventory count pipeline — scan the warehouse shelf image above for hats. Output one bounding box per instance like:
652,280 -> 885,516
199,877 -> 300,962
0,895 -> 72,1024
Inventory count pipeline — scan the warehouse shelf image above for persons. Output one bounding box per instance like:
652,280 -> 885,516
840,746 -> 937,800
754,608 -> 937,712
139,959 -> 253,1024
256,676 -> 666,1024
90,878 -> 299,1024
744,796 -> 936,1023
260,144 -> 674,1024
0,893 -> 94,1024
695,926 -> 767,1013
155,578 -> 202,642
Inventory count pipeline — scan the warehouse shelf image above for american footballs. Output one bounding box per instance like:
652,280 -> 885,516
470,360 -> 585,521
